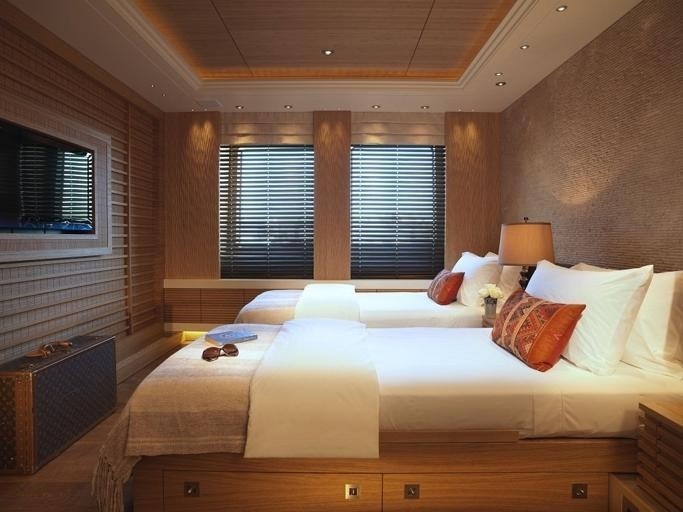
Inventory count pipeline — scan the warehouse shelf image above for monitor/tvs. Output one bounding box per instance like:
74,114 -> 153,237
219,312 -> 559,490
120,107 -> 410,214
0,117 -> 97,234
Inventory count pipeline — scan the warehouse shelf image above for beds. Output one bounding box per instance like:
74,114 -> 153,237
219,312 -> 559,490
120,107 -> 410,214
235,263 -> 575,327
90,271 -> 683,512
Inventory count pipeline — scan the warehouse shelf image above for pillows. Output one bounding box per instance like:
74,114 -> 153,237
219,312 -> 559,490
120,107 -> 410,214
484,297 -> 496,319
567,262 -> 683,373
484,251 -> 523,301
427,269 -> 464,306
525,260 -> 653,376
490,289 -> 587,372
450,251 -> 503,306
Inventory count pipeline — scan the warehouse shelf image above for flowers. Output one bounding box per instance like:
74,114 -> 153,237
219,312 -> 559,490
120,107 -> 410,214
477,283 -> 504,299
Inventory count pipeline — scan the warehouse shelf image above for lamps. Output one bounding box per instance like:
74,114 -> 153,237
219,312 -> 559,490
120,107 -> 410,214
498,216 -> 556,292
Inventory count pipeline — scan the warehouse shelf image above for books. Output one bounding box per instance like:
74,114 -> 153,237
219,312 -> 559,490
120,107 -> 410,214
204,330 -> 258,347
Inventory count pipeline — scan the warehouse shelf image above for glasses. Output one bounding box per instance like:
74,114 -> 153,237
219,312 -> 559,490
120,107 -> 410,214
202,342 -> 239,361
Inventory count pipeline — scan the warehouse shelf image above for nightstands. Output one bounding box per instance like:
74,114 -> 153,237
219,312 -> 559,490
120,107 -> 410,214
610,399 -> 683,512
